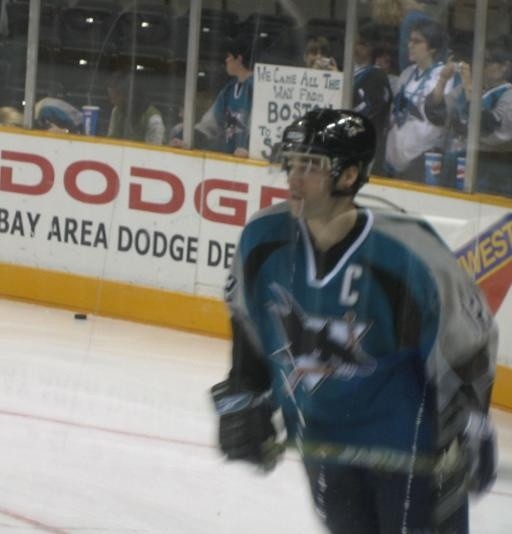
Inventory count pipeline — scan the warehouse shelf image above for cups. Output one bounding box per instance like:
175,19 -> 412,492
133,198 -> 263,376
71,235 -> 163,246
82,105 -> 100,134
425,152 -> 442,185
455,157 -> 465,189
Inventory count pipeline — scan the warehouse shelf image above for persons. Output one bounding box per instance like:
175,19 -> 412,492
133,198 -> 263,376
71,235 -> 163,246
211,109 -> 496,534
0,1 -> 512,200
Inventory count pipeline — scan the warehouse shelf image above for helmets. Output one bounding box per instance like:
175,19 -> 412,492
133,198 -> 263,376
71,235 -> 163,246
276,107 -> 375,197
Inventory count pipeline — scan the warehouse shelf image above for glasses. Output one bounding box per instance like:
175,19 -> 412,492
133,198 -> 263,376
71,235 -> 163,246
408,38 -> 425,44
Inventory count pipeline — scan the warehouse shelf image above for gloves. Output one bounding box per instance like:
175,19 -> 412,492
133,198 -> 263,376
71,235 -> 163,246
211,378 -> 277,463
